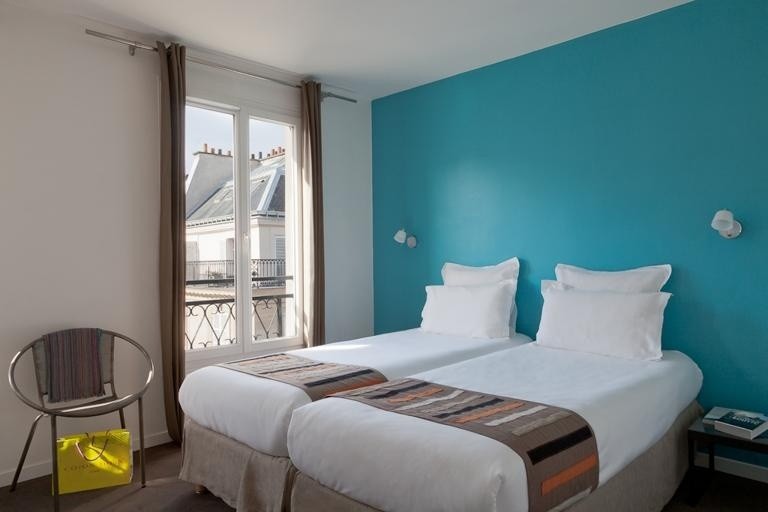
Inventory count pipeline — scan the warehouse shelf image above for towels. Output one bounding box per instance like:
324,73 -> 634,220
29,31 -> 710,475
42,329 -> 106,403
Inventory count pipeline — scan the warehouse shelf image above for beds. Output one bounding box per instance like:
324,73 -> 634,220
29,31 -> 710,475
177,328 -> 534,512
287,340 -> 705,512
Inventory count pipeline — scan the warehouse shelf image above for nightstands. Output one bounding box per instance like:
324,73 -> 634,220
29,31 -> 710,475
688,416 -> 768,507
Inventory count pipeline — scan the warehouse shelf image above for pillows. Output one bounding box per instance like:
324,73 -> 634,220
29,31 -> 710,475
555,263 -> 673,292
536,279 -> 673,360
420,279 -> 515,342
441,258 -> 520,313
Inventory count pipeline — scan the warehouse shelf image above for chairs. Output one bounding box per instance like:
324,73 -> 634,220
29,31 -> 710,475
7,327 -> 154,512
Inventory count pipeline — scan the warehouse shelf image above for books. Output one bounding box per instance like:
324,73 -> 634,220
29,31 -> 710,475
701,405 -> 767,440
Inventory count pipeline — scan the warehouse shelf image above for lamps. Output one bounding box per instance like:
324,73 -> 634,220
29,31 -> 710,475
711,208 -> 742,239
394,229 -> 416,248
